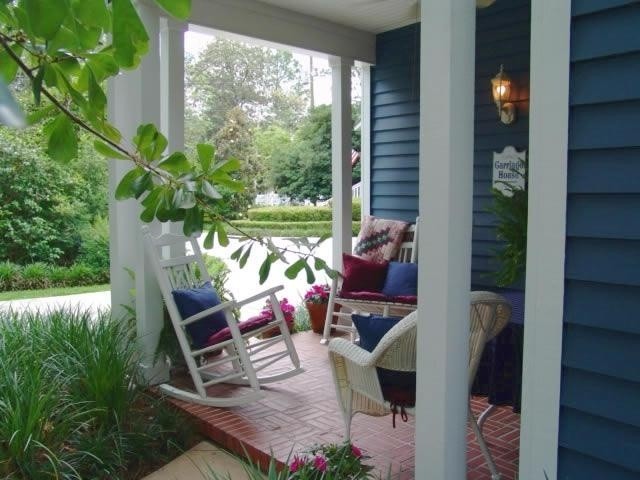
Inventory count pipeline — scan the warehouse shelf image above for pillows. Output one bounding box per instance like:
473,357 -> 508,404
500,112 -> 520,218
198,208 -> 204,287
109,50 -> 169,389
383,261 -> 416,296
339,251 -> 386,292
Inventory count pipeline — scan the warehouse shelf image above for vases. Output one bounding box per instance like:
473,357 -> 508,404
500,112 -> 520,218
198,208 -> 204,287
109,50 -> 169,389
306,303 -> 343,333
262,319 -> 293,339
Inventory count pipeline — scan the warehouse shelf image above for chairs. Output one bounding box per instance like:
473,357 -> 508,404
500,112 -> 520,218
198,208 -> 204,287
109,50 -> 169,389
318,221 -> 417,351
323,290 -> 508,480
142,222 -> 305,409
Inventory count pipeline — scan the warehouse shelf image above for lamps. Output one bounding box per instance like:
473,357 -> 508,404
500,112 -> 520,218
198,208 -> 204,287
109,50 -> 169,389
490,64 -> 518,125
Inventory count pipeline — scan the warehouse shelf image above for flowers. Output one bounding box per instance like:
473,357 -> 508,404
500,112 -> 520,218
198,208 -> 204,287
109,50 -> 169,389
286,433 -> 370,476
303,283 -> 339,303
259,298 -> 293,313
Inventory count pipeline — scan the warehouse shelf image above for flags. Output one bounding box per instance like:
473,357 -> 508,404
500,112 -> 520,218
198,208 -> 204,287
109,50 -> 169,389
352,149 -> 359,165
